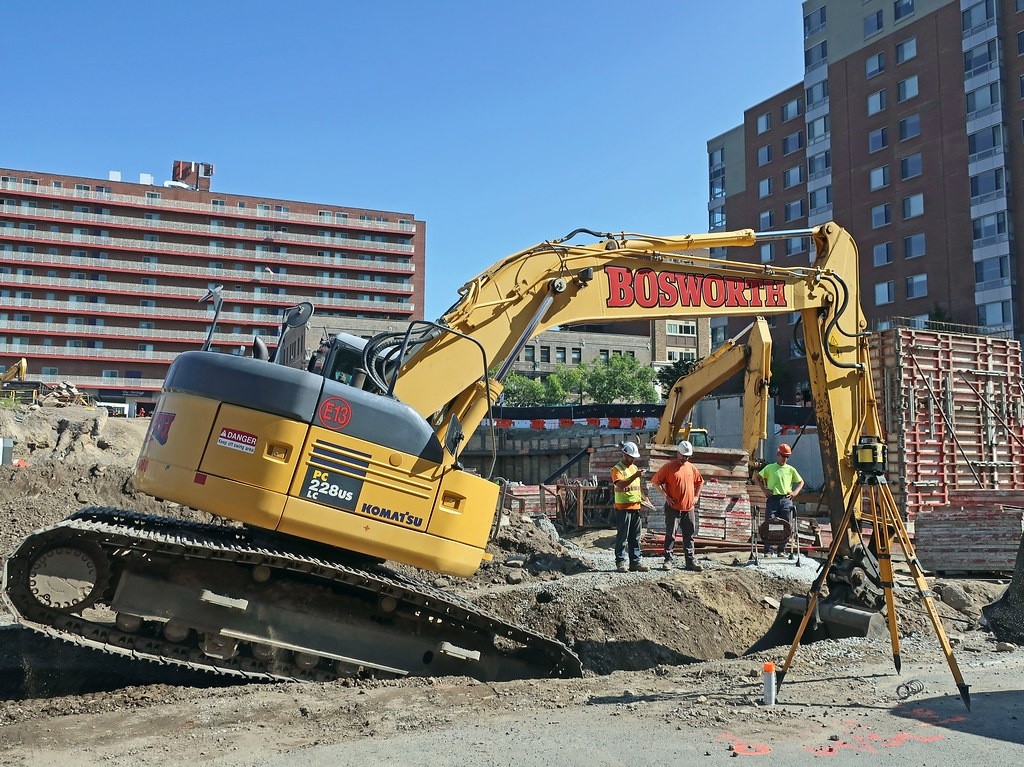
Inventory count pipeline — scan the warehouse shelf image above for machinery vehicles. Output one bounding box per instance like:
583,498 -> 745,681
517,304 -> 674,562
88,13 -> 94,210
648,316 -> 773,478
0,221 -> 890,686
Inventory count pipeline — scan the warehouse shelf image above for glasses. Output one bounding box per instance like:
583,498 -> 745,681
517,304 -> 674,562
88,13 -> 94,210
682,455 -> 689,457
627,454 -> 634,458
779,453 -> 789,458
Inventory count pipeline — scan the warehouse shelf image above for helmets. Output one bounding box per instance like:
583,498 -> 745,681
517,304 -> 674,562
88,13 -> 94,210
778,443 -> 791,455
678,440 -> 693,456
621,442 -> 640,457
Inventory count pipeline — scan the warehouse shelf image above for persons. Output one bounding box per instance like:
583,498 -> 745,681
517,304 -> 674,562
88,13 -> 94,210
755,444 -> 804,558
651,440 -> 704,571
611,441 -> 654,573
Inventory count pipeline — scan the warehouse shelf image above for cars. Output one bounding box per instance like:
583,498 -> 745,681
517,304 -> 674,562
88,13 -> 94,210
105,406 -> 116,417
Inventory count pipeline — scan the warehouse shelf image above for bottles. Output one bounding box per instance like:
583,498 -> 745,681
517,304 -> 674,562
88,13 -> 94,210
763,662 -> 775,704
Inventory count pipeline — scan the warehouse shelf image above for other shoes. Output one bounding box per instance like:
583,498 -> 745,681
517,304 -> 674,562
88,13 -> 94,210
777,552 -> 785,557
618,565 -> 627,572
630,564 -> 650,571
765,551 -> 772,557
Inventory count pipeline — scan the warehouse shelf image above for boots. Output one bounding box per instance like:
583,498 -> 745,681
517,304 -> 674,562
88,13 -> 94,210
663,558 -> 672,570
685,559 -> 703,571
